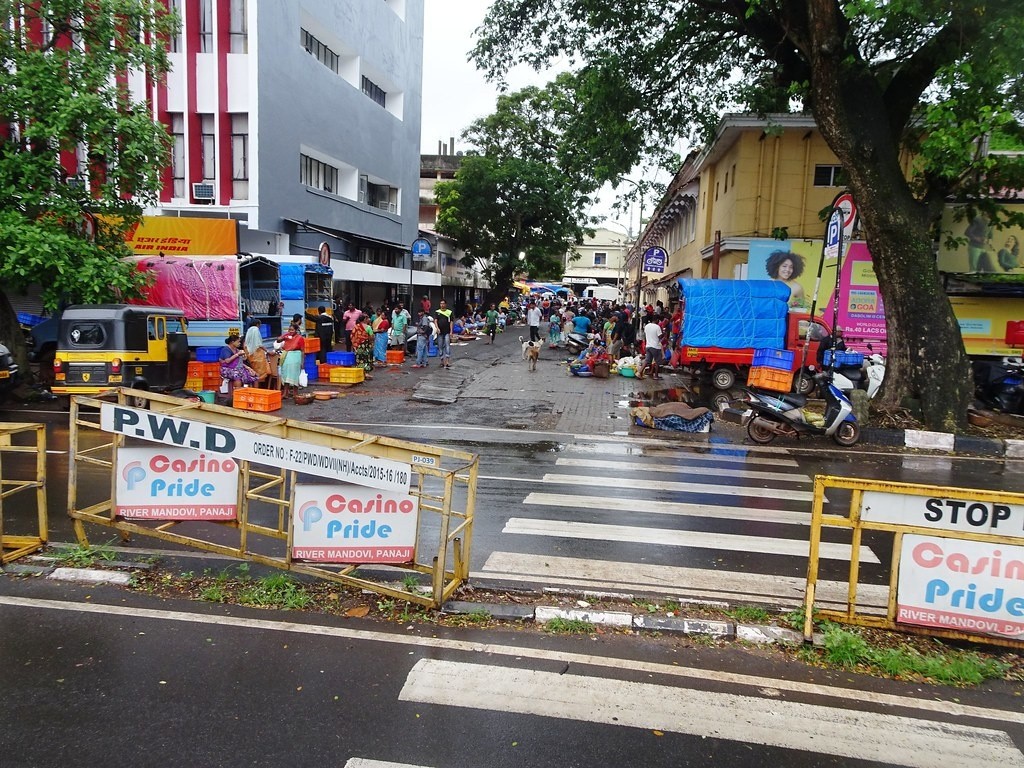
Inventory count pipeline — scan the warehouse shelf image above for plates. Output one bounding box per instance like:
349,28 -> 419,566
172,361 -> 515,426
242,352 -> 245,353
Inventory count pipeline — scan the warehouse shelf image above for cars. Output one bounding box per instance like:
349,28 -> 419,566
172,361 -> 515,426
0,344 -> 20,386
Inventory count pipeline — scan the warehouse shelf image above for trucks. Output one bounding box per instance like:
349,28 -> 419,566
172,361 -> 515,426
681,312 -> 838,390
587,287 -> 624,300
113,252 -> 336,349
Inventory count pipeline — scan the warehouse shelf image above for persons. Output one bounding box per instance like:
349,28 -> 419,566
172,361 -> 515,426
410,308 -> 429,369
964,205 -> 1023,272
434,298 -> 454,368
242,318 -> 276,390
420,295 -> 431,316
765,249 -> 807,299
305,306 -> 334,365
485,303 -> 499,345
817,325 -> 846,400
335,298 -> 412,364
219,334 -> 267,391
453,294 -> 675,380
291,314 -> 306,337
349,316 -> 377,381
527,301 -> 546,343
277,324 -> 305,402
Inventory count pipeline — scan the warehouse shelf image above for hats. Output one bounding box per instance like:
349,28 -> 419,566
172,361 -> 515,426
836,325 -> 845,332
869,354 -> 884,364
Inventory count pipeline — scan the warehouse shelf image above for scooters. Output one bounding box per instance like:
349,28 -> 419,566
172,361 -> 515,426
567,330 -> 590,354
735,365 -> 860,445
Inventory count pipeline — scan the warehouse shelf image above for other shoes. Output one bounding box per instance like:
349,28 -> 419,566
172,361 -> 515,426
440,363 -> 443,368
365,374 -> 373,379
635,372 -> 644,380
411,364 -> 420,368
445,364 -> 451,368
654,377 -> 663,380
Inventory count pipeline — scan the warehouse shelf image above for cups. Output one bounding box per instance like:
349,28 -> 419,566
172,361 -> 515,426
288,336 -> 292,339
240,350 -> 244,352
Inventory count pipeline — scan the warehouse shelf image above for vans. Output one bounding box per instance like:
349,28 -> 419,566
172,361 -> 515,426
50,302 -> 189,408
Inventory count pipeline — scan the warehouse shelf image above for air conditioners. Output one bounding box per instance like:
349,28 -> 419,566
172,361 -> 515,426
192,183 -> 215,200
64,175 -> 86,196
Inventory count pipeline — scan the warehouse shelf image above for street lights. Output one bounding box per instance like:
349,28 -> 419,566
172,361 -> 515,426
617,175 -> 642,334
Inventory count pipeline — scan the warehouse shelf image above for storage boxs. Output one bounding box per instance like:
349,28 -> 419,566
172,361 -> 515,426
179,307 -> 366,412
747,347 -> 796,393
385,350 -> 405,364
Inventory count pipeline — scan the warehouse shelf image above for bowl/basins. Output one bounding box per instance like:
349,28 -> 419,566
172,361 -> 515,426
295,395 -> 316,405
577,372 -> 592,377
314,392 -> 340,400
450,338 -> 459,343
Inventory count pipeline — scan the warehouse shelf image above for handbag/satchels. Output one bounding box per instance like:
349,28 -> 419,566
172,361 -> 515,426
299,369 -> 309,387
